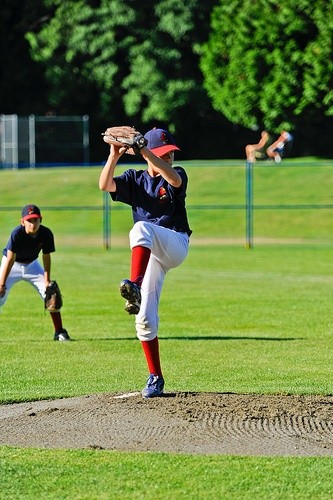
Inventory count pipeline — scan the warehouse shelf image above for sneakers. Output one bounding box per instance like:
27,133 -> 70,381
119,278 -> 141,315
141,373 -> 165,400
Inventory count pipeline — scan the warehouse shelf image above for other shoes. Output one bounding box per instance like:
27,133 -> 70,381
54,329 -> 71,342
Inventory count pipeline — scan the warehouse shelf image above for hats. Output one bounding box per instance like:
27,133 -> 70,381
141,127 -> 181,158
20,204 -> 41,223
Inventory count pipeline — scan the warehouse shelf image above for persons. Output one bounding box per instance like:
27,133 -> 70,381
245,130 -> 273,163
0,204 -> 71,341
99,126 -> 192,399
266,129 -> 294,162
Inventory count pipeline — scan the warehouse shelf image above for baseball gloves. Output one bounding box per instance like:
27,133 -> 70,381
103,125 -> 148,150
44,280 -> 63,312
